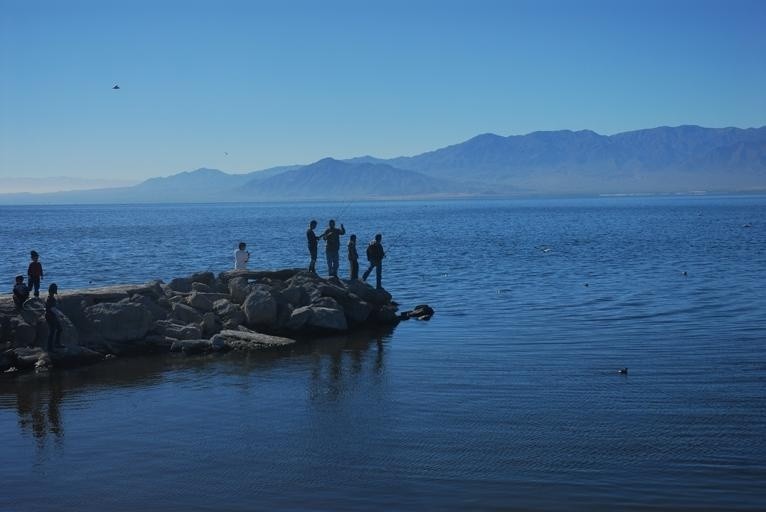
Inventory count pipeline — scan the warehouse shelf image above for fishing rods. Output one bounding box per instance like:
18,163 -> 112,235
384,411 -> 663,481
318,195 -> 356,238
384,223 -> 410,257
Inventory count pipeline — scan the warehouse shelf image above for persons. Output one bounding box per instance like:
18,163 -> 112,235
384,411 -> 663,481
234,242 -> 250,272
307,219 -> 324,274
348,234 -> 362,281
323,219 -> 345,278
26,250 -> 43,298
45,283 -> 66,352
12,275 -> 30,308
361,234 -> 385,289
